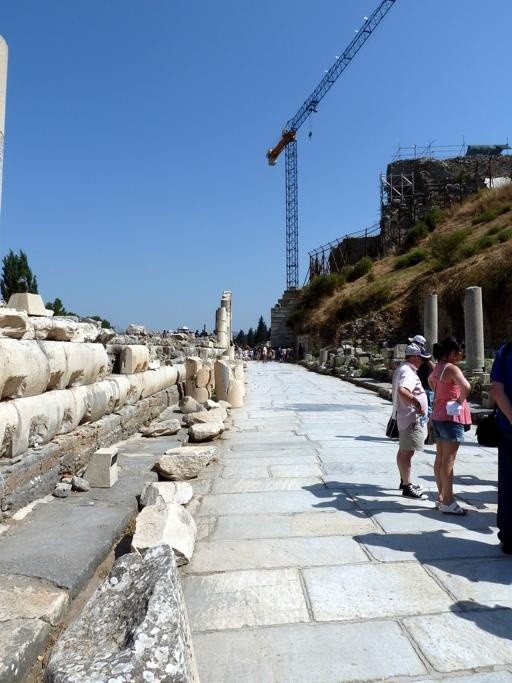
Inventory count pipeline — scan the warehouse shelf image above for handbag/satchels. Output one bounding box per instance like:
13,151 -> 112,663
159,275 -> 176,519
476,412 -> 499,447
386,414 -> 399,438
426,421 -> 437,444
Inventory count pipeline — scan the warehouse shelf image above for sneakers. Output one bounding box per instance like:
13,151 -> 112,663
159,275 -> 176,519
399,478 -> 429,500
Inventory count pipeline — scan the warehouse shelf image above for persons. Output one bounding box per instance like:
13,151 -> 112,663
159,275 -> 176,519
488,336 -> 510,556
161,327 -> 216,339
236,341 -> 294,364
391,341 -> 432,500
427,335 -> 473,514
408,334 -> 437,445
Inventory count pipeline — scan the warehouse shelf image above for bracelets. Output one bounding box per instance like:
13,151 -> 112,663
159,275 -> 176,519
415,402 -> 421,407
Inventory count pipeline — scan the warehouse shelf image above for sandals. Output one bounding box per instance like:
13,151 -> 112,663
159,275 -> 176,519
435,500 -> 468,515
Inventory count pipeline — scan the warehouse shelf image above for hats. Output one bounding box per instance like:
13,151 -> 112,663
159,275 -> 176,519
404,335 -> 432,358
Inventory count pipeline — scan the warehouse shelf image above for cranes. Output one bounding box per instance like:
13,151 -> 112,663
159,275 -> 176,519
266,0 -> 396,290
